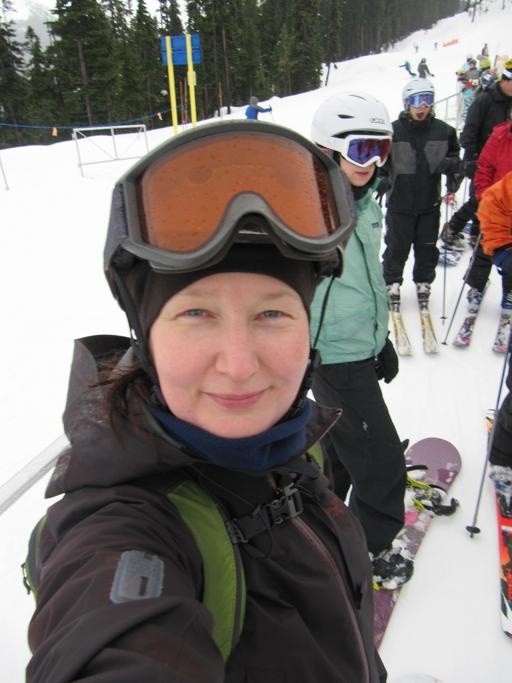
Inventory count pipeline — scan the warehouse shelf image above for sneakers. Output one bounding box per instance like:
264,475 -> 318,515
415,281 -> 430,309
500,290 -> 512,319
365,543 -> 414,586
490,465 -> 512,518
467,288 -> 483,315
440,221 -> 466,252
385,281 -> 400,312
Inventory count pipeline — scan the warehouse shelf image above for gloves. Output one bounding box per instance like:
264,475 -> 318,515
438,158 -> 468,193
374,177 -> 392,200
376,330 -> 398,383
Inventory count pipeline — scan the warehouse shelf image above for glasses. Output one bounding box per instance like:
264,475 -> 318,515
104,119 -> 358,276
310,124 -> 393,168
403,93 -> 434,108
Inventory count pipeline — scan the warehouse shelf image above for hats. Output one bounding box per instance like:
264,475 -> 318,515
108,246 -> 325,346
501,58 -> 512,81
250,96 -> 257,104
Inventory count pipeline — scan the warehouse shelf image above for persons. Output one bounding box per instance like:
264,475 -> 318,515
244,96 -> 271,119
305,92 -> 415,589
375,77 -> 463,303
418,57 -> 434,79
23,118 -> 392,683
475,167 -> 512,501
398,60 -> 416,76
455,41 -> 512,131
441,58 -> 511,248
462,105 -> 512,317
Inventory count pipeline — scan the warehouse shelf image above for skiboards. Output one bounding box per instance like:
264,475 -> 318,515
486,409 -> 512,637
391,299 -> 440,356
452,277 -> 512,353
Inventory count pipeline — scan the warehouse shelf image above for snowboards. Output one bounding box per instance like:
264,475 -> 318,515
367,438 -> 461,650
438,223 -> 472,266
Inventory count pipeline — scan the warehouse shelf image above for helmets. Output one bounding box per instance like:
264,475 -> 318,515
402,78 -> 436,112
309,89 -> 394,168
467,56 -> 499,85
